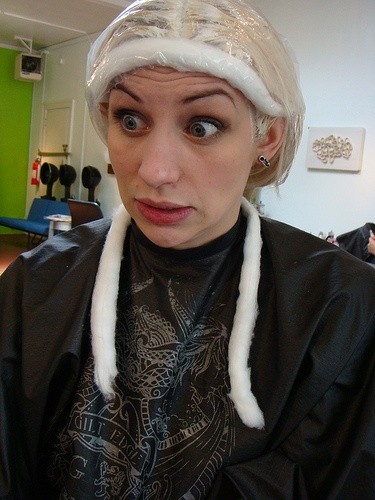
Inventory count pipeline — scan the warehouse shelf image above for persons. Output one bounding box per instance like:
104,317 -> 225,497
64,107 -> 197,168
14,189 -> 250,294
2,0 -> 375,500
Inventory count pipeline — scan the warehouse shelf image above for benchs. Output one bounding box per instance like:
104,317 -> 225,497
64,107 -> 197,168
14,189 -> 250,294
0,198 -> 72,249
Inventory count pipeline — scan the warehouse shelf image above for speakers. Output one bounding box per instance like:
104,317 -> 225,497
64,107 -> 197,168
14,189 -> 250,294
15,54 -> 43,82
